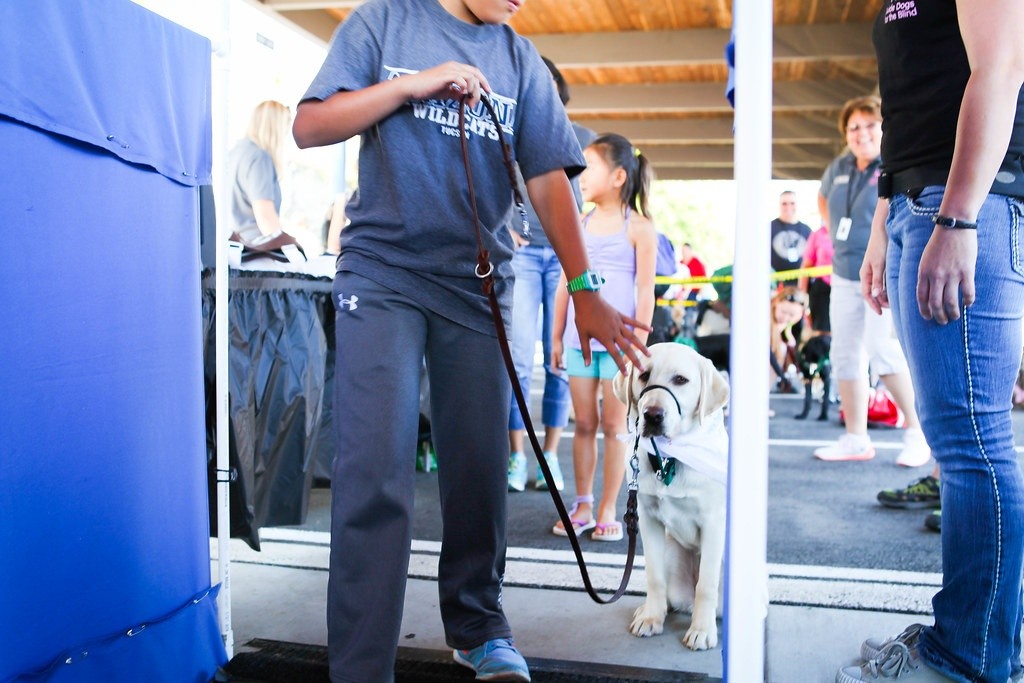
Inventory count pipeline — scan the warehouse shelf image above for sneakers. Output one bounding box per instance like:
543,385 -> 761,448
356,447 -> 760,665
835,623 -> 1023,683
814,433 -> 876,462
876,473 -> 945,509
536,452 -> 563,491
454,632 -> 530,683
926,504 -> 942,533
896,429 -> 932,467
506,455 -> 528,491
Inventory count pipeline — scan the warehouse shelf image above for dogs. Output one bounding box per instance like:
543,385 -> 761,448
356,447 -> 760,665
794,334 -> 831,422
613,341 -> 729,650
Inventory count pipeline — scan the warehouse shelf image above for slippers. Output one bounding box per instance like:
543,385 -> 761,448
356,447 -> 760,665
554,517 -> 597,535
592,522 -> 624,542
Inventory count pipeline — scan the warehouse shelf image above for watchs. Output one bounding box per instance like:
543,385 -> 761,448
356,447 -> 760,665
565,269 -> 603,294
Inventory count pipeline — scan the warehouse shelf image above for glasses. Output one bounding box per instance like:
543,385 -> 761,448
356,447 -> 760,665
846,121 -> 883,132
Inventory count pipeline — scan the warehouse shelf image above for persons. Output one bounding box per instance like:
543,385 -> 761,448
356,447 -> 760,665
798,190 -> 836,337
548,134 -> 657,541
835,0 -> 1024,683
770,286 -> 809,386
770,191 -> 813,286
814,94 -> 930,467
507,56 -> 599,491
291,0 -> 653,683
229,100 -> 293,242
663,242 -> 707,310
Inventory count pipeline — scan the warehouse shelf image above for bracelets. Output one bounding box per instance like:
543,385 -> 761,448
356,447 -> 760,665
932,215 -> 978,229
